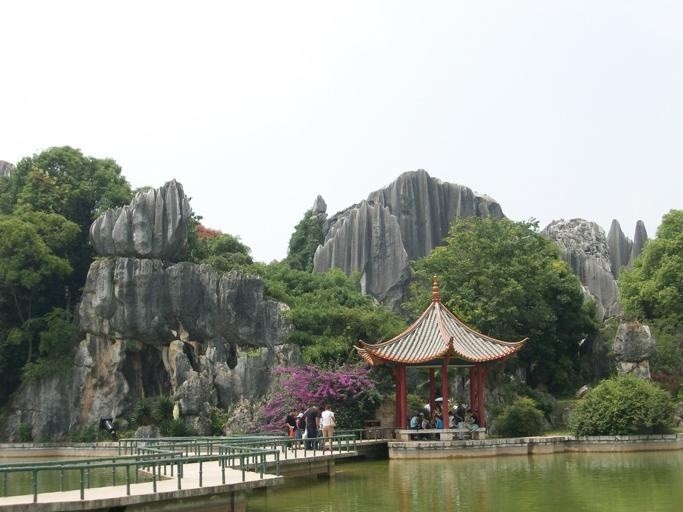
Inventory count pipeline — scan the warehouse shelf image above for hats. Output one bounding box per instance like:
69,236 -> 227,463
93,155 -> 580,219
296,412 -> 304,419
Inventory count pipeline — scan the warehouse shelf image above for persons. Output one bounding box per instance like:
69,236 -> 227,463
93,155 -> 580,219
285,402 -> 338,452
410,400 -> 485,442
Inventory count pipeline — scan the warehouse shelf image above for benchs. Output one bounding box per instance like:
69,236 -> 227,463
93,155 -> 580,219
393,427 -> 487,442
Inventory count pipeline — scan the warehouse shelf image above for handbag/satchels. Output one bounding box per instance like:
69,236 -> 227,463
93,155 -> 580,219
328,416 -> 337,427
